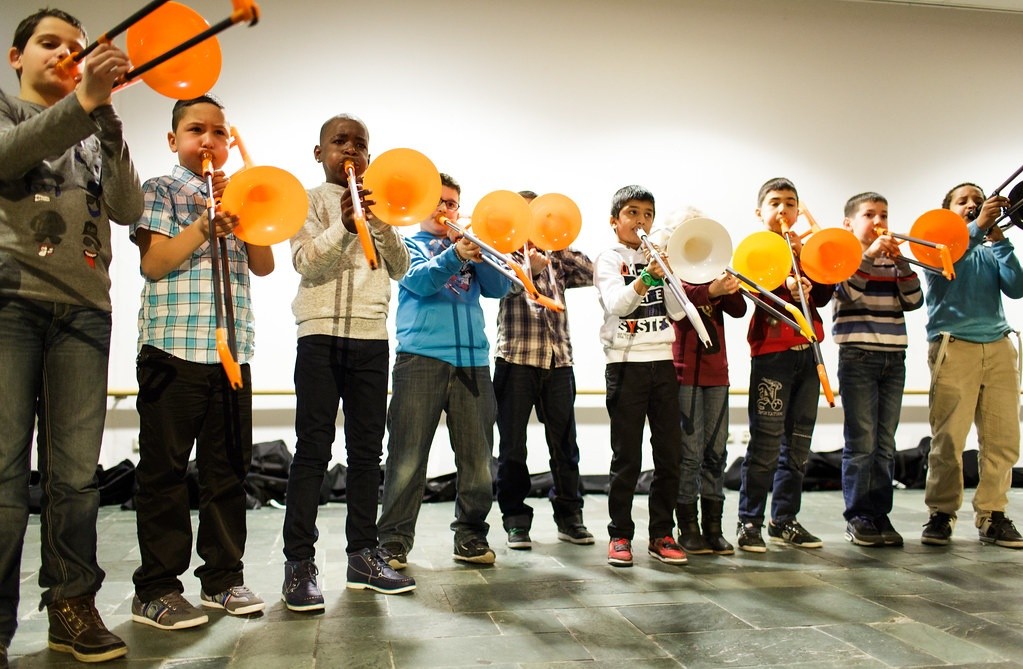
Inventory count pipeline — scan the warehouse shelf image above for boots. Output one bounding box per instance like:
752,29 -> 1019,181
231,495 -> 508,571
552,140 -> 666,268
701,499 -> 734,554
675,502 -> 713,554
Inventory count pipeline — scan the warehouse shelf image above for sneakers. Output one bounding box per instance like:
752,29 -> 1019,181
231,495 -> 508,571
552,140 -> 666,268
48,595 -> 127,661
452,540 -> 496,564
557,523 -> 594,544
736,516 -> 767,553
845,515 -> 884,547
870,514 -> 904,547
346,548 -> 417,595
379,545 -> 408,570
767,517 -> 823,547
506,526 -> 532,548
132,589 -> 209,630
281,557 -> 324,610
648,537 -> 689,565
608,538 -> 633,564
200,586 -> 265,615
921,512 -> 956,544
978,511 -> 1023,547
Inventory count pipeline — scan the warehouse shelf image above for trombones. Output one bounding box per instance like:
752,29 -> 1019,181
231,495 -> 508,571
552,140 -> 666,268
779,199 -> 862,408
200,125 -> 309,391
967,164 -> 1023,243
725,231 -> 818,343
872,209 -> 969,281
344,148 -> 441,271
523,193 -> 582,312
436,191 -> 540,300
56,0 -> 259,100
633,217 -> 732,349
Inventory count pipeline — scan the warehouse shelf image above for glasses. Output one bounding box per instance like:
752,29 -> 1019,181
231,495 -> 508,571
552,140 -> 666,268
437,198 -> 460,211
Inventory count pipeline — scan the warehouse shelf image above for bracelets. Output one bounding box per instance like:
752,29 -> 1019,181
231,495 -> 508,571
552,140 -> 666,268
640,267 -> 665,287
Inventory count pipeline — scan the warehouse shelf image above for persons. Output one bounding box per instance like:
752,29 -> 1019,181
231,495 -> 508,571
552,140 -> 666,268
594,185 -> 690,566
1,10 -> 144,668
672,273 -> 747,555
280,115 -> 411,611
921,183 -> 1023,548
128,95 -> 276,631
375,173 -> 511,571
833,192 -> 924,546
734,177 -> 836,551
492,190 -> 594,549
